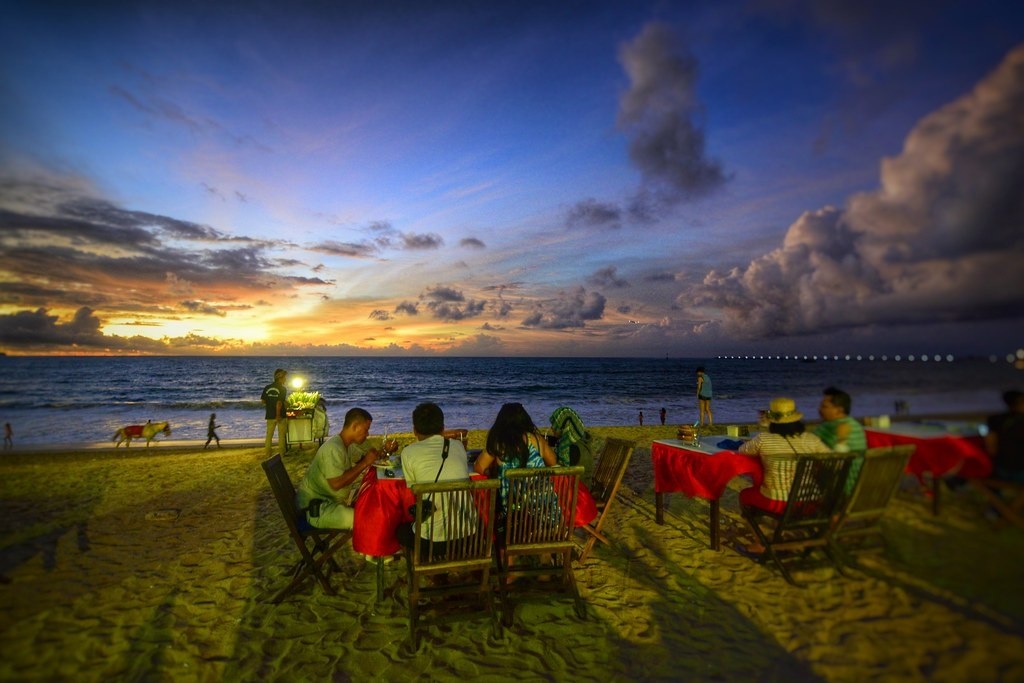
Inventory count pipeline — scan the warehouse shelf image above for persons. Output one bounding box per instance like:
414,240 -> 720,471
659,408 -> 666,425
4,423 -> 14,449
474,402 -> 561,584
812,387 -> 868,526
204,413 -> 222,450
549,406 -> 593,528
400,402 -> 477,610
696,366 -> 712,427
261,369 -> 289,458
294,408 -> 399,565
147,420 -> 151,424
738,397 -> 840,558
639,412 -> 643,426
894,400 -> 909,416
984,390 -> 1024,532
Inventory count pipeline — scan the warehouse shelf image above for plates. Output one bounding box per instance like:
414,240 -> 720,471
372,459 -> 402,469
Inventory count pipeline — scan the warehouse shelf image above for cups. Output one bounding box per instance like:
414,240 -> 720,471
455,436 -> 469,451
386,440 -> 398,455
692,432 -> 700,448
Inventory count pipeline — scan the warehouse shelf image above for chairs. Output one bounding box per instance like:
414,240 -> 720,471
259,430 -> 637,656
733,445 -> 1024,587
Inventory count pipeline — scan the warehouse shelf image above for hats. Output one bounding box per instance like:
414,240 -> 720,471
274,369 -> 288,379
766,397 -> 803,424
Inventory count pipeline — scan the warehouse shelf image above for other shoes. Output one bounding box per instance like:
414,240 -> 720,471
367,552 -> 393,565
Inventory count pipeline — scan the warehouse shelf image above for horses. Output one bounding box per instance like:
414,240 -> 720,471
111,421 -> 171,448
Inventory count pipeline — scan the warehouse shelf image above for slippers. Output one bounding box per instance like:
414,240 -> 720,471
777,548 -> 787,556
736,542 -> 767,562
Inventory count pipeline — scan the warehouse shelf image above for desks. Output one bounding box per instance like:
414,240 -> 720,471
651,431 -> 765,552
861,422 -> 993,518
352,449 -> 599,604
